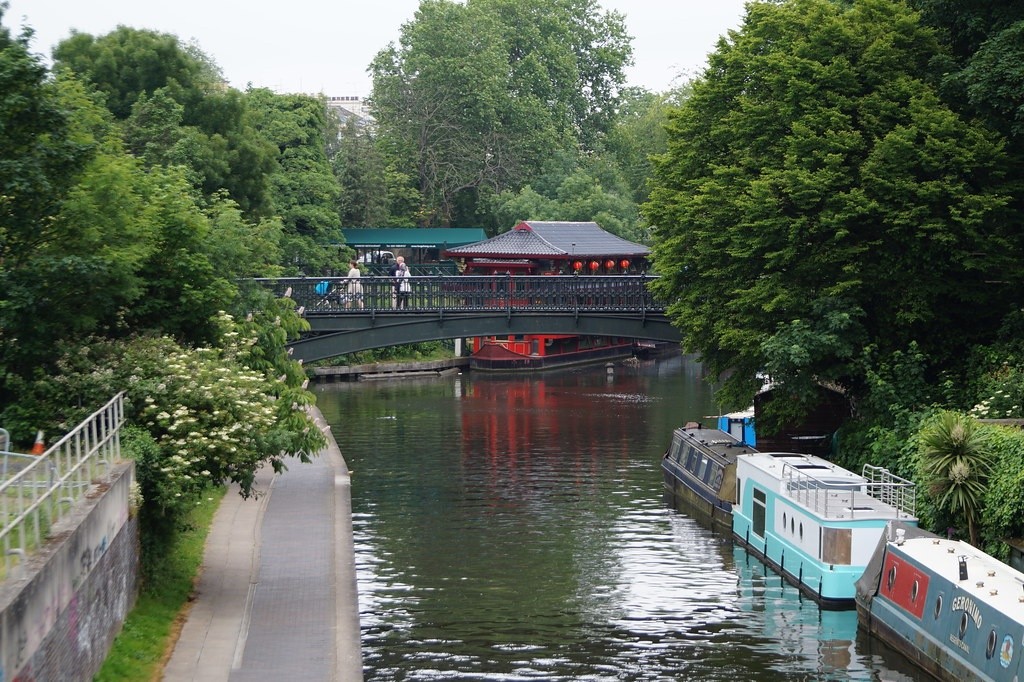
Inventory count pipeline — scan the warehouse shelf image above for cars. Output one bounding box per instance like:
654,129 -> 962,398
358,250 -> 397,265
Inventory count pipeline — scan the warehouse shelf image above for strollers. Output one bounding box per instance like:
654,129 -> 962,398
313,280 -> 347,311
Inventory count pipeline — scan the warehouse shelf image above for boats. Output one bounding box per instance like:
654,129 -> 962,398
853,521 -> 1023,682
659,420 -> 760,528
729,451 -> 922,609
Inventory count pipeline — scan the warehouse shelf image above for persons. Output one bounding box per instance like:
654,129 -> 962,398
390,256 -> 412,309
340,260 -> 363,309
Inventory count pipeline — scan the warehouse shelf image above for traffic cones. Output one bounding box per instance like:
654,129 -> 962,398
30,428 -> 47,456
